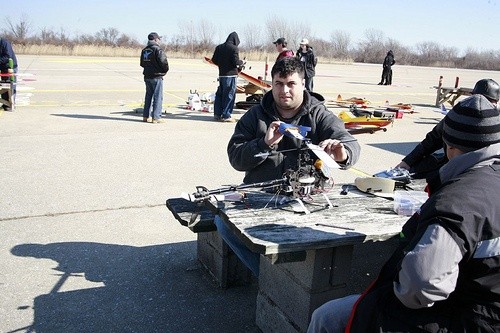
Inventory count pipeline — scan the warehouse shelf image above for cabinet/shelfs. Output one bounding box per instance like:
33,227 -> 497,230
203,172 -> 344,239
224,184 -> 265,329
0,71 -> 17,112
434,85 -> 474,106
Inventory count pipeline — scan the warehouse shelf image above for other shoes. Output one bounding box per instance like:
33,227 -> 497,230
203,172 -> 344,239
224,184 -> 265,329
143,117 -> 165,123
213,114 -> 236,122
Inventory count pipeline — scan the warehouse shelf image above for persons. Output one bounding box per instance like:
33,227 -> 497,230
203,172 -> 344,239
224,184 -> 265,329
0,37 -> 18,110
211,32 -> 242,121
140,31 -> 169,123
303,95 -> 500,333
272,38 -> 294,63
377,49 -> 394,85
227,54 -> 362,181
294,38 -> 316,92
392,78 -> 500,178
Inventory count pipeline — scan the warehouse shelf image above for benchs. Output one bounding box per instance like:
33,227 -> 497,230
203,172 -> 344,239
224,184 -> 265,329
194,201 -> 254,289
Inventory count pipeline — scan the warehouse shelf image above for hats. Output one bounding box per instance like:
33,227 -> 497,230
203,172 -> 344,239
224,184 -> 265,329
442,94 -> 500,152
148,32 -> 162,40
300,39 -> 309,45
273,38 -> 288,45
469,79 -> 500,102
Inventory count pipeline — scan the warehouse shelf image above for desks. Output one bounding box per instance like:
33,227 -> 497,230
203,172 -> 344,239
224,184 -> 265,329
218,184 -> 432,333
